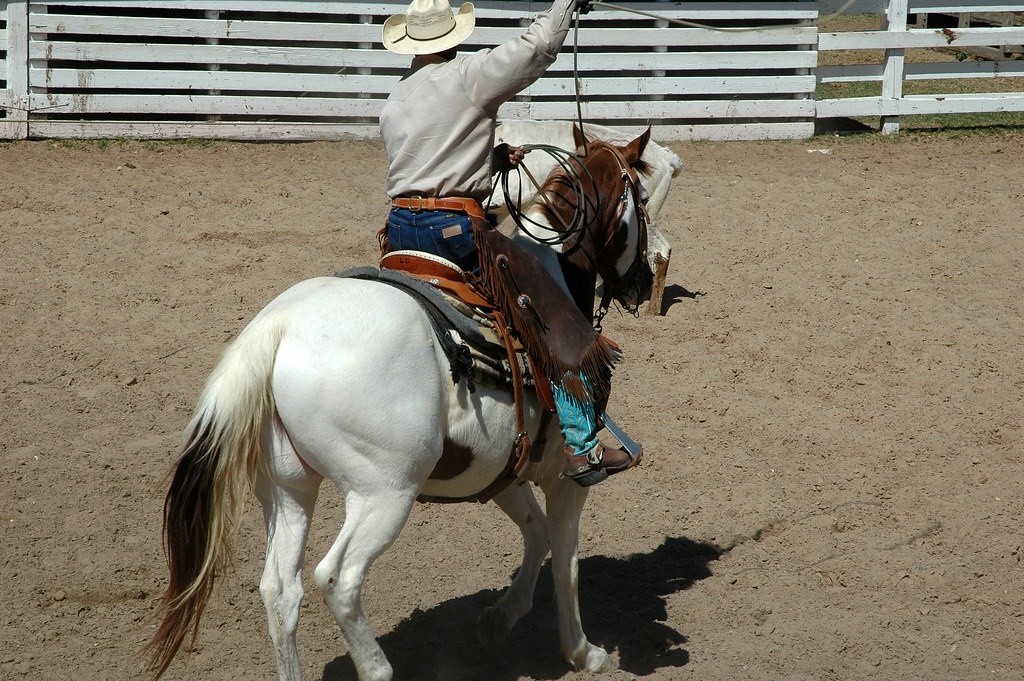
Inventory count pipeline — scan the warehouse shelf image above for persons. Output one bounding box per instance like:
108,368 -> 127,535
378,0 -> 642,489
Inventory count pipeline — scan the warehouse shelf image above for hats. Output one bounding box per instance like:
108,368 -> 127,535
381,0 -> 476,55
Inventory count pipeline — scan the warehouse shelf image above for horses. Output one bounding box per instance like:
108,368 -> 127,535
133,121 -> 683,681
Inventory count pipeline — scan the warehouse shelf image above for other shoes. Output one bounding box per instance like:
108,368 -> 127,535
564,440 -> 644,486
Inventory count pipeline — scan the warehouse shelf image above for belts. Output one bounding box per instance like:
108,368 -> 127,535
389,196 -> 469,211
